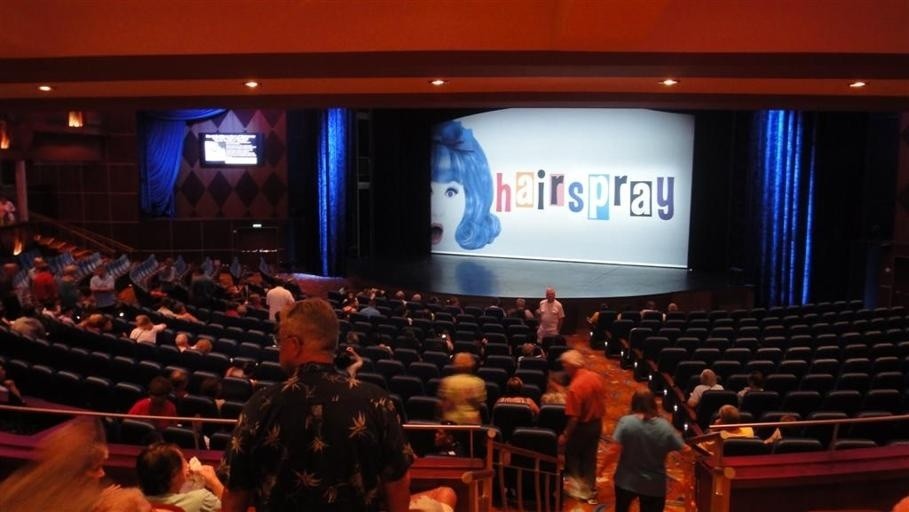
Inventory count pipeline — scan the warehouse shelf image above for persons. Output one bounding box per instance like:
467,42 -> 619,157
611,390 -> 691,512
430,124 -> 504,252
424,419 -> 470,459
438,341 -> 607,504
709,405 -> 757,446
688,369 -> 726,407
224,298 -> 417,512
587,301 -> 677,323
0,197 -> 17,225
535,289 -> 565,344
1,413 -> 224,512
1,257 -> 303,386
336,284 -> 463,381
127,370 -> 224,429
487,296 -> 533,319
736,371 -> 765,399
761,412 -> 799,444
1,368 -> 26,407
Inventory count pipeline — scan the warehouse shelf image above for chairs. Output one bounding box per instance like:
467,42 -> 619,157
3,250 -> 581,510
588,250 -> 907,509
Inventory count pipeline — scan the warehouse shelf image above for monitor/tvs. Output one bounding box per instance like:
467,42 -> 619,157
199,132 -> 265,168
